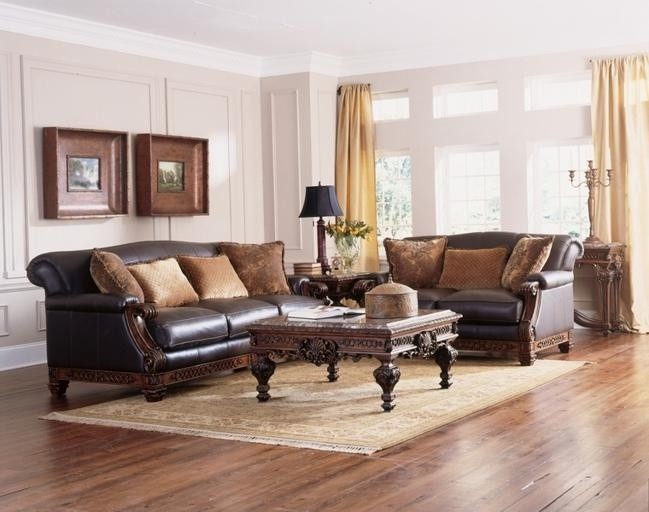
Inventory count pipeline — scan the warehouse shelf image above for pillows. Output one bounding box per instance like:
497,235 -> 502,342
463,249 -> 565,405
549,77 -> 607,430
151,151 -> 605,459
437,247 -> 508,290
501,235 -> 554,288
89,247 -> 144,303
126,257 -> 199,307
217,241 -> 294,296
383,236 -> 448,288
177,252 -> 248,300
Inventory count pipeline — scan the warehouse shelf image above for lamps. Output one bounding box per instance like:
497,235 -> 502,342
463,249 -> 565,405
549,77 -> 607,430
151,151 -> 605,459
298,181 -> 343,272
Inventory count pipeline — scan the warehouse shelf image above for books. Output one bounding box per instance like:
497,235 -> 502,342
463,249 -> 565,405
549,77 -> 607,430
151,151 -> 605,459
288,305 -> 365,319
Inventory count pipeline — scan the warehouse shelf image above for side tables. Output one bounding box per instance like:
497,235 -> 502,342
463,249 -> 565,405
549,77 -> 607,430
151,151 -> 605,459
288,272 -> 388,307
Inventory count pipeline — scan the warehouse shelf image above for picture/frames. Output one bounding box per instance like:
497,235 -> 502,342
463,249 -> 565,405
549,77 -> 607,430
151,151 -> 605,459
157,159 -> 185,192
67,155 -> 101,192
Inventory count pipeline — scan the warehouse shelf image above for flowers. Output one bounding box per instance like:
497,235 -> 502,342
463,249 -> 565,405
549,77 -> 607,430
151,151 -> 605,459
324,216 -> 373,243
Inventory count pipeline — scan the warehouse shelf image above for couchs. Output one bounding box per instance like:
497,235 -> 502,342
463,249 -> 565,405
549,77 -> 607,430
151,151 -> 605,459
26,240 -> 334,402
353,232 -> 584,366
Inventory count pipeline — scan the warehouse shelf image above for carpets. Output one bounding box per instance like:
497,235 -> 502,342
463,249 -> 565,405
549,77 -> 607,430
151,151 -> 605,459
37,356 -> 596,455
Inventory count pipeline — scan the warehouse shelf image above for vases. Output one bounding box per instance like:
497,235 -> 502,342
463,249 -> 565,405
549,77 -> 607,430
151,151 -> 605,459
336,234 -> 360,274
